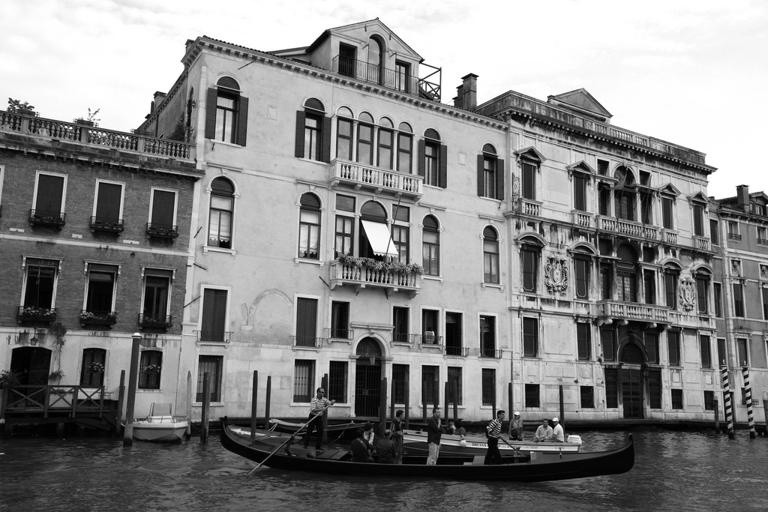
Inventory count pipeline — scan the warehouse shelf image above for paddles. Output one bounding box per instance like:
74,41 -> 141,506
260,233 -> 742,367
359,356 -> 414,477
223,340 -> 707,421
246,402 -> 333,477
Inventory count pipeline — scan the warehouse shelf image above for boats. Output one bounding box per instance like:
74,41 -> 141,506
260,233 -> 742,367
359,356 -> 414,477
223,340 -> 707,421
122,416 -> 190,444
220,420 -> 634,482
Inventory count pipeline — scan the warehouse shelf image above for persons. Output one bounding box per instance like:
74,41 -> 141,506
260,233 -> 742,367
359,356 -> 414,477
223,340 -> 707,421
447,419 -> 456,433
533,419 -> 553,441
508,411 -> 524,440
371,428 -> 399,462
545,417 -> 565,443
303,387 -> 336,450
484,410 -> 506,465
454,422 -> 465,435
359,422 -> 377,462
388,410 -> 404,464
350,427 -> 377,463
426,407 -> 442,465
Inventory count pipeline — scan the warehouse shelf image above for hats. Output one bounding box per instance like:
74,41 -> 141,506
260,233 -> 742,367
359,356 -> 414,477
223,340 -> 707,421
514,412 -> 520,416
552,418 -> 559,422
